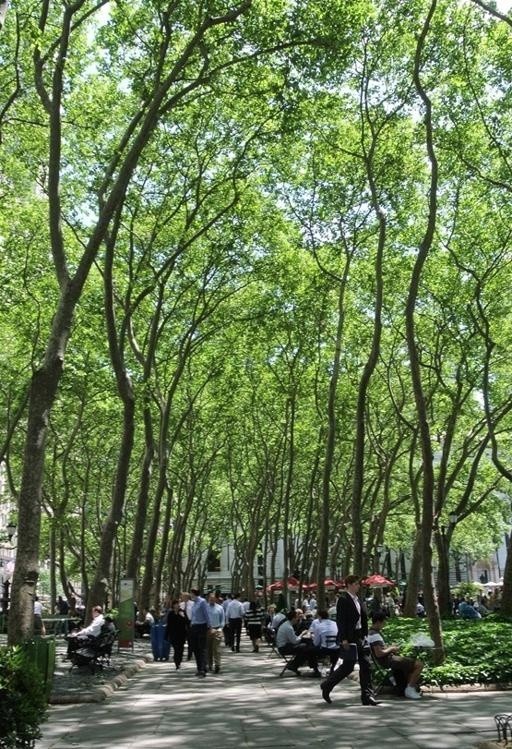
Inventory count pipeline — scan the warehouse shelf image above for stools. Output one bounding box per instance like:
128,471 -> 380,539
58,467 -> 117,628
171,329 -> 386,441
277,653 -> 301,675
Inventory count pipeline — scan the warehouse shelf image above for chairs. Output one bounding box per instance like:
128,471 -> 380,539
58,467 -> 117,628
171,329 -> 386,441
322,636 -> 340,677
264,628 -> 280,658
366,639 -> 397,698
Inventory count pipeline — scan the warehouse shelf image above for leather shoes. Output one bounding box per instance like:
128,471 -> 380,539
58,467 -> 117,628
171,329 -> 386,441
320,684 -> 332,704
364,699 -> 381,706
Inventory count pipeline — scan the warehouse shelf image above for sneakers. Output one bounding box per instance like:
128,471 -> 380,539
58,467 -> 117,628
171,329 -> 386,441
224,641 -> 258,653
287,665 -> 301,674
195,666 -> 220,678
404,686 -> 421,699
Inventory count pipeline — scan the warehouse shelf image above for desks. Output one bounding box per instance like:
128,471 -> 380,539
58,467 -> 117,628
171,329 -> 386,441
412,646 -> 444,695
41,617 -> 82,638
302,635 -> 313,643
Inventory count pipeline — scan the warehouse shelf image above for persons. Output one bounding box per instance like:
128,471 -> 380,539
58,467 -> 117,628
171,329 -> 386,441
453,573 -> 502,619
382,592 -> 426,618
34,583 -> 115,662
320,576 -> 380,705
366,613 -> 425,699
135,589 -> 340,676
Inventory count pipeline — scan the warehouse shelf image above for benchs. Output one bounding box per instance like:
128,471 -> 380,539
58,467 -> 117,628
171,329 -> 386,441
69,629 -> 122,674
